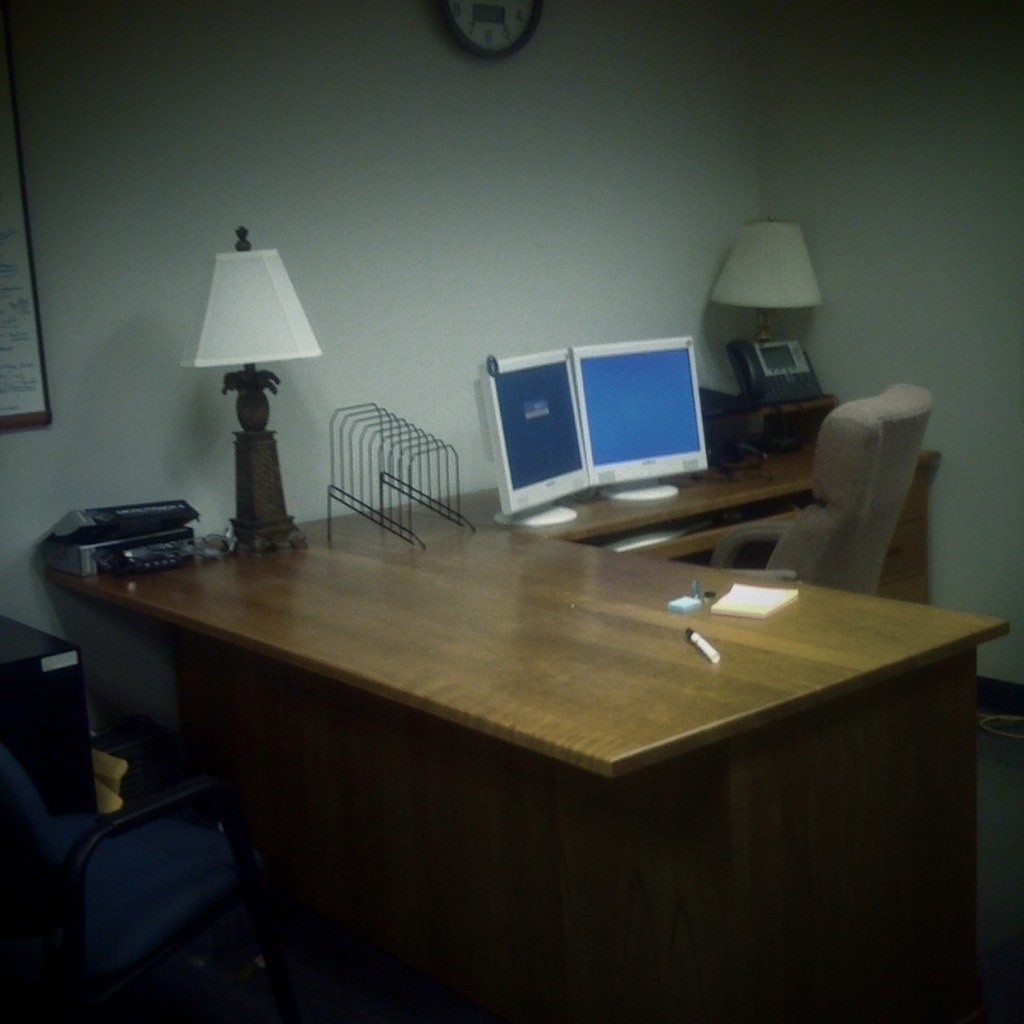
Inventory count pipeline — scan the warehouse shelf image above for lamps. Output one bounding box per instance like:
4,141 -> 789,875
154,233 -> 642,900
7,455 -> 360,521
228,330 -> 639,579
710,215 -> 822,339
187,228 -> 324,554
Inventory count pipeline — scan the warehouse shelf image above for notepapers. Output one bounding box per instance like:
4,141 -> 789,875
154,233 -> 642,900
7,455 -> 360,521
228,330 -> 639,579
711,583 -> 799,620
668,595 -> 703,614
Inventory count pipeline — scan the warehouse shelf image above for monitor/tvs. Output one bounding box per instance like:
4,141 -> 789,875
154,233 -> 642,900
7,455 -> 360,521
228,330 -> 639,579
484,337 -> 710,526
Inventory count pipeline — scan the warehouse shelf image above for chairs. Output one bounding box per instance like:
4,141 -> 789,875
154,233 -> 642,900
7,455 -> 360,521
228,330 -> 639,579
710,384 -> 933,591
1,709 -> 298,1024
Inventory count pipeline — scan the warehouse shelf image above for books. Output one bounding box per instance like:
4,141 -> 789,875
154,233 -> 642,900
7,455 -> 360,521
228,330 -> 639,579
710,583 -> 799,619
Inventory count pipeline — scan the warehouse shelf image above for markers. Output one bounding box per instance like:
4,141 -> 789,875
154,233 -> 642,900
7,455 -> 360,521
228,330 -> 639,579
685,627 -> 721,664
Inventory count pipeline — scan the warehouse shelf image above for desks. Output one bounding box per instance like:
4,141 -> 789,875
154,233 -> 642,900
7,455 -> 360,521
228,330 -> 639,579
50,437 -> 1009,1024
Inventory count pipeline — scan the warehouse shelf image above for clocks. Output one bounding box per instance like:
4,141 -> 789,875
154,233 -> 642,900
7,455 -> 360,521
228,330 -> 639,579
431,0 -> 544,60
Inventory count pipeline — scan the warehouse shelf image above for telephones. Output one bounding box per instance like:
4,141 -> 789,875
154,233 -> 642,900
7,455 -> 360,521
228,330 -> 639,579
725,329 -> 823,402
